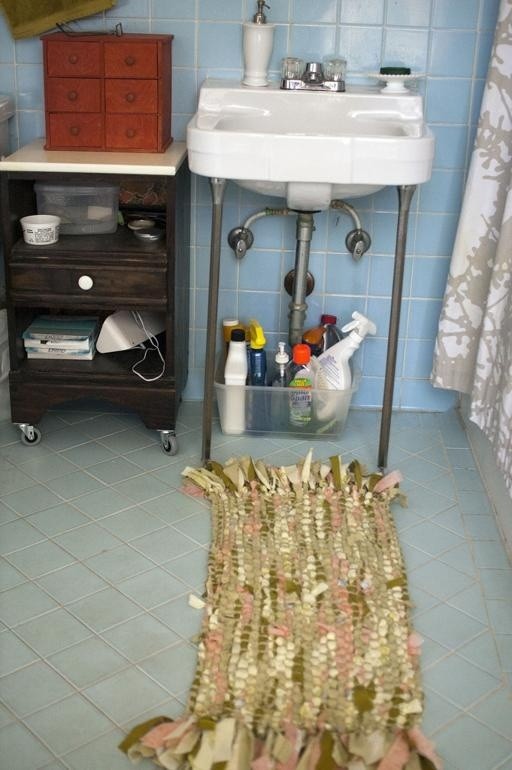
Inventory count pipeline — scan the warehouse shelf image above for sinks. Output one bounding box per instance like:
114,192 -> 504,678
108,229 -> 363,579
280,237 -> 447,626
185,77 -> 436,212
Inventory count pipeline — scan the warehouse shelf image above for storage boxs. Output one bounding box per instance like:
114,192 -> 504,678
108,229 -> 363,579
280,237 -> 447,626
30,175 -> 121,236
212,343 -> 363,442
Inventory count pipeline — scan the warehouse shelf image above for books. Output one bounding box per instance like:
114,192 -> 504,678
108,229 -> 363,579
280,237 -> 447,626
22,315 -> 99,360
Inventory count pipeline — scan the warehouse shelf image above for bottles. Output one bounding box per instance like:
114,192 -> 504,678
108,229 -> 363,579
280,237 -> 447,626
220,318 -> 239,356
286,343 -> 316,429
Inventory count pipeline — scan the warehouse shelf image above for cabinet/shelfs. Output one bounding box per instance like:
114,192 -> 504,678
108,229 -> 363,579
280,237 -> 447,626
0,140 -> 192,457
39,30 -> 175,154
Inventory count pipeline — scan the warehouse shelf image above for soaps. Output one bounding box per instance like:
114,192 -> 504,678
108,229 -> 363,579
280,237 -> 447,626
380,67 -> 412,75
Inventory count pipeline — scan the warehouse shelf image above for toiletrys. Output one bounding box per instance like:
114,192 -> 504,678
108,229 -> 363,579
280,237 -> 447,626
242,0 -> 275,87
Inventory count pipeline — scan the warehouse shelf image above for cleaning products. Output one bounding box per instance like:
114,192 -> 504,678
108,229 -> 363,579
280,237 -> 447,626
222,316 -> 249,358
313,312 -> 376,422
248,317 -> 268,433
284,343 -> 318,433
224,328 -> 249,434
271,342 -> 288,387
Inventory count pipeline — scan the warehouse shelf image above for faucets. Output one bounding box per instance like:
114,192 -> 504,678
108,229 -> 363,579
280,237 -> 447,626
305,62 -> 324,87
